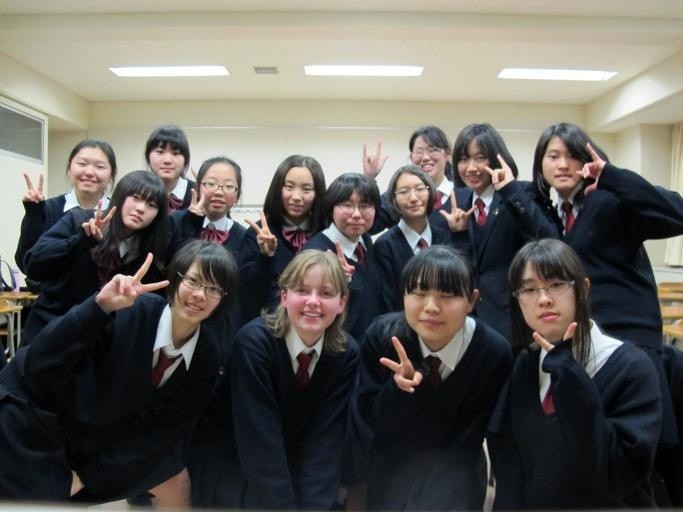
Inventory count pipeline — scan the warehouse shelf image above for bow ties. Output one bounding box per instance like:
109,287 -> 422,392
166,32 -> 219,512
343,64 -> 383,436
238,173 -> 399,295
164,194 -> 182,213
200,227 -> 229,245
281,230 -> 312,250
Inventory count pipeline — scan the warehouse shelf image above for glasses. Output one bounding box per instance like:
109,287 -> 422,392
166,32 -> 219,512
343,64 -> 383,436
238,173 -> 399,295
172,269 -> 228,298
200,180 -> 239,194
512,278 -> 580,302
394,185 -> 431,197
338,201 -> 373,212
411,146 -> 443,155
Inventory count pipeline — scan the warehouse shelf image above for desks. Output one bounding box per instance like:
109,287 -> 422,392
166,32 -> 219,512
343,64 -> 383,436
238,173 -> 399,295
0,291 -> 38,363
657,282 -> 683,348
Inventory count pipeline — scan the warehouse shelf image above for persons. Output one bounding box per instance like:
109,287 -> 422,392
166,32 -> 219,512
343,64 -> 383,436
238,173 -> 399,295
0,121 -> 682,511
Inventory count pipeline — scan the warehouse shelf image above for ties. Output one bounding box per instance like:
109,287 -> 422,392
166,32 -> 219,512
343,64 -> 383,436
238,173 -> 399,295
354,241 -> 367,262
423,356 -> 443,383
473,197 -> 490,225
560,202 -> 576,234
541,380 -> 554,413
152,346 -> 183,388
294,351 -> 313,387
417,239 -> 429,251
433,192 -> 442,208
